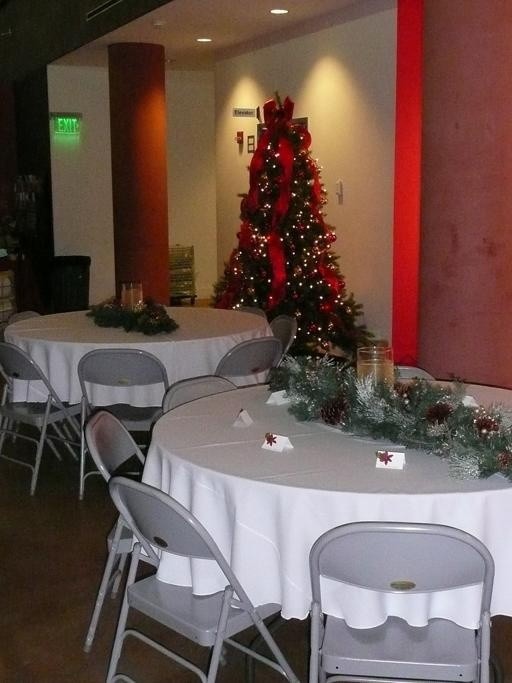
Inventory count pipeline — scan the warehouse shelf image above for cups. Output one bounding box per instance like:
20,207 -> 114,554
355,345 -> 395,389
118,278 -> 144,310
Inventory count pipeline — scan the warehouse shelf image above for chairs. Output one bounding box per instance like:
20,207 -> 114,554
82,413 -> 175,653
107,476 -> 300,682
307,521 -> 500,683
391,366 -> 434,379
0,305 -> 297,505
